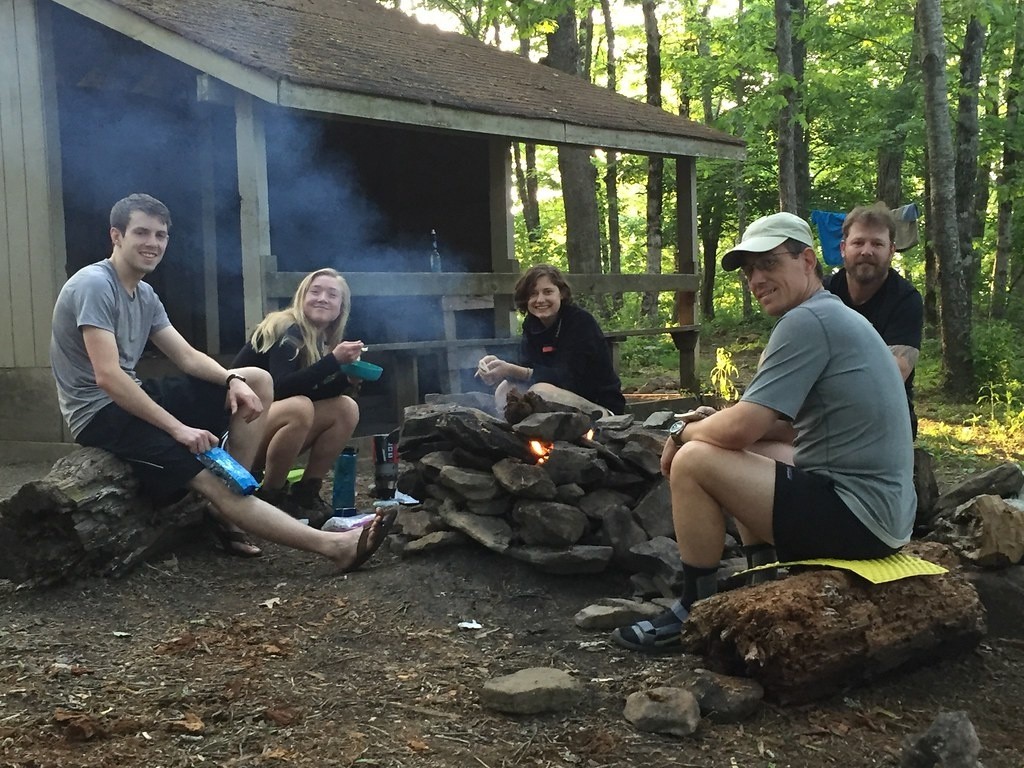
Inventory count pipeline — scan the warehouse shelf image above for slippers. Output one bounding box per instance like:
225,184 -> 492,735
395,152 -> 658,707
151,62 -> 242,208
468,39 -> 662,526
203,507 -> 260,559
342,507 -> 398,571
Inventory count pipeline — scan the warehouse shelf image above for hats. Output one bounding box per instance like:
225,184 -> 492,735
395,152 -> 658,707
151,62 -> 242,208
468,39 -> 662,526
721,212 -> 815,272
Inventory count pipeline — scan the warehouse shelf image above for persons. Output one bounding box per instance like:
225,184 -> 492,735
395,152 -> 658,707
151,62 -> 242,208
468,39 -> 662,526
610,212 -> 917,653
822,204 -> 923,443
49,193 -> 396,573
473,264 -> 626,417
228,267 -> 364,519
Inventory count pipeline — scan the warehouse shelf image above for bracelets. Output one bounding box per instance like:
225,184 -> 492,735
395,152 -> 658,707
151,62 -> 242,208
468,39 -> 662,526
226,373 -> 246,390
524,367 -> 530,381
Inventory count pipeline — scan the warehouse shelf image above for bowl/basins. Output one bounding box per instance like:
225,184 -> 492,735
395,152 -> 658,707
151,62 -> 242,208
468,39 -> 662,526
340,360 -> 383,382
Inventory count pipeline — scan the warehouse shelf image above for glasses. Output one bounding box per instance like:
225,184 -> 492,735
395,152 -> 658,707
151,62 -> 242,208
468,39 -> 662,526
744,247 -> 799,281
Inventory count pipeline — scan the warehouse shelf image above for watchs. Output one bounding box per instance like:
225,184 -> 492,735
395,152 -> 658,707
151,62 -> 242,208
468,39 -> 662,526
670,420 -> 687,449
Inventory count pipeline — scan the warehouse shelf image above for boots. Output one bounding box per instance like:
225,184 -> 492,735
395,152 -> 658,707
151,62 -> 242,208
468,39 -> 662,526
296,479 -> 334,521
257,482 -> 323,526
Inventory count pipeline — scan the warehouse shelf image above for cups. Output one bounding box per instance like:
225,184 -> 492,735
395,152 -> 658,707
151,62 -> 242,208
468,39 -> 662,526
371,433 -> 398,501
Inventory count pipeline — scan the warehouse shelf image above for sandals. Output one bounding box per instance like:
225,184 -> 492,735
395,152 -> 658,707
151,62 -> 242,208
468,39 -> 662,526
612,596 -> 690,651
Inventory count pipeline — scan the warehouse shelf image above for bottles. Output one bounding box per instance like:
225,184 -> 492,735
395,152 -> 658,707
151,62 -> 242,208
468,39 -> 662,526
331,446 -> 358,518
